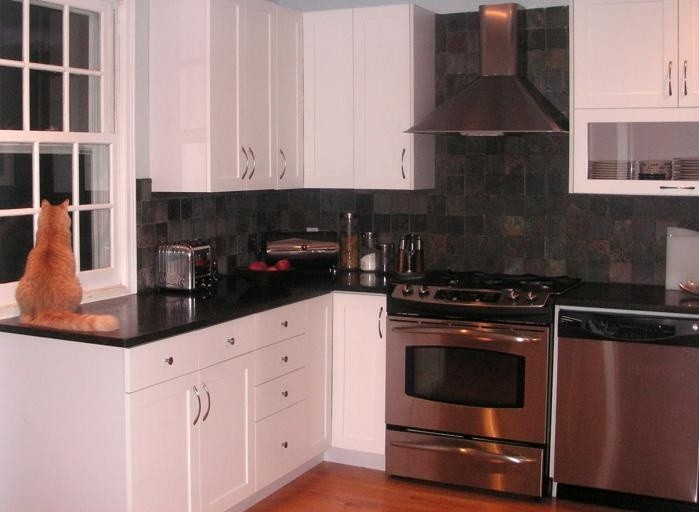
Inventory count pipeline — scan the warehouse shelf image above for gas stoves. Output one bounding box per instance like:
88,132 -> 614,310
387,269 -> 581,315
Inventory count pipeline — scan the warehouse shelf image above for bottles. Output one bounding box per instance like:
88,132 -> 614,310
339,212 -> 424,279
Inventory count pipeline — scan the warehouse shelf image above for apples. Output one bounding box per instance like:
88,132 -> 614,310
248,259 -> 292,272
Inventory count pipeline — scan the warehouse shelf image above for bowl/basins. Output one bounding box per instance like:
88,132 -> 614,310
638,158 -> 674,180
233,268 -> 294,288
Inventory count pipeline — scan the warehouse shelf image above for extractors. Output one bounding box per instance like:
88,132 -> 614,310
404,2 -> 568,139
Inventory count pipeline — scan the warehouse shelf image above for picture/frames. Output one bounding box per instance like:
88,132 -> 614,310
277,4 -> 304,191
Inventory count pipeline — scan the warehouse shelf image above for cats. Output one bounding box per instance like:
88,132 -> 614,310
15,198 -> 119,333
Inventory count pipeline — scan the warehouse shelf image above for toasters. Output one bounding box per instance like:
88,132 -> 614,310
157,295 -> 199,326
155,238 -> 220,291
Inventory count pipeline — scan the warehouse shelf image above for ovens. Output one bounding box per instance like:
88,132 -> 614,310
386,312 -> 549,500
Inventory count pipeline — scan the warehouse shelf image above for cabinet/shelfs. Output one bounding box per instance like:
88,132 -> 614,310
149,0 -> 277,193
254,293 -> 332,505
0,314 -> 254,512
568,0 -> 699,196
305,4 -> 436,190
332,290 -> 386,471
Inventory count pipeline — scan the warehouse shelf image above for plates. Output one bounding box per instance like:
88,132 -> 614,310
673,158 -> 699,179
587,159 -> 637,180
679,275 -> 699,302
